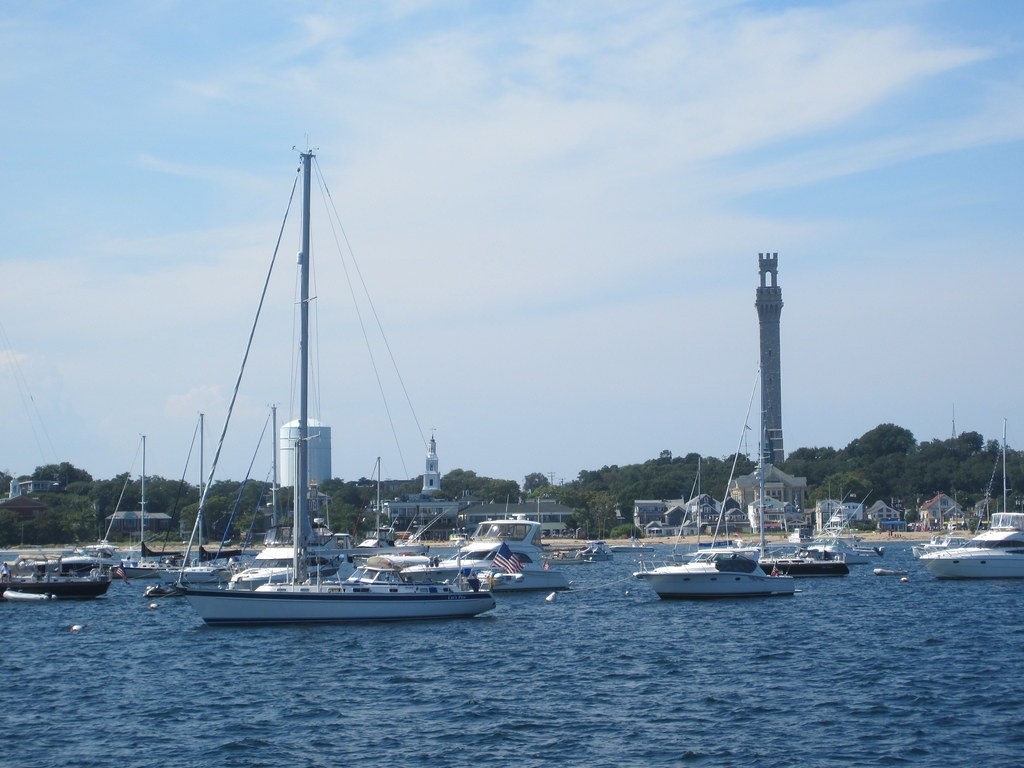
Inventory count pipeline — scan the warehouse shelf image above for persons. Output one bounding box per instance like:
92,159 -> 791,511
679,531 -> 740,538
887,529 -> 892,538
0,563 -> 11,579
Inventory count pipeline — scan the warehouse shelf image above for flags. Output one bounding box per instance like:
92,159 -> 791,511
114,565 -> 128,583
914,512 -> 929,524
770,561 -> 779,577
493,542 -> 526,574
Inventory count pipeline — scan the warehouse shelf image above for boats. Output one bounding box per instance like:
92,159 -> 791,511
576,537 -> 614,561
756,543 -> 850,577
807,538 -> 869,565
395,514 -> 572,593
789,527 -> 814,543
911,534 -> 967,560
541,547 -> 596,564
847,535 -> 878,557
0,557 -> 117,606
609,536 -> 655,553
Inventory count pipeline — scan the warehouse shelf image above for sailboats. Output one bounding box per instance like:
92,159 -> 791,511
632,363 -> 798,600
918,417 -> 1024,579
169,149 -> 497,624
664,455 -> 703,563
23,402 -> 282,584
